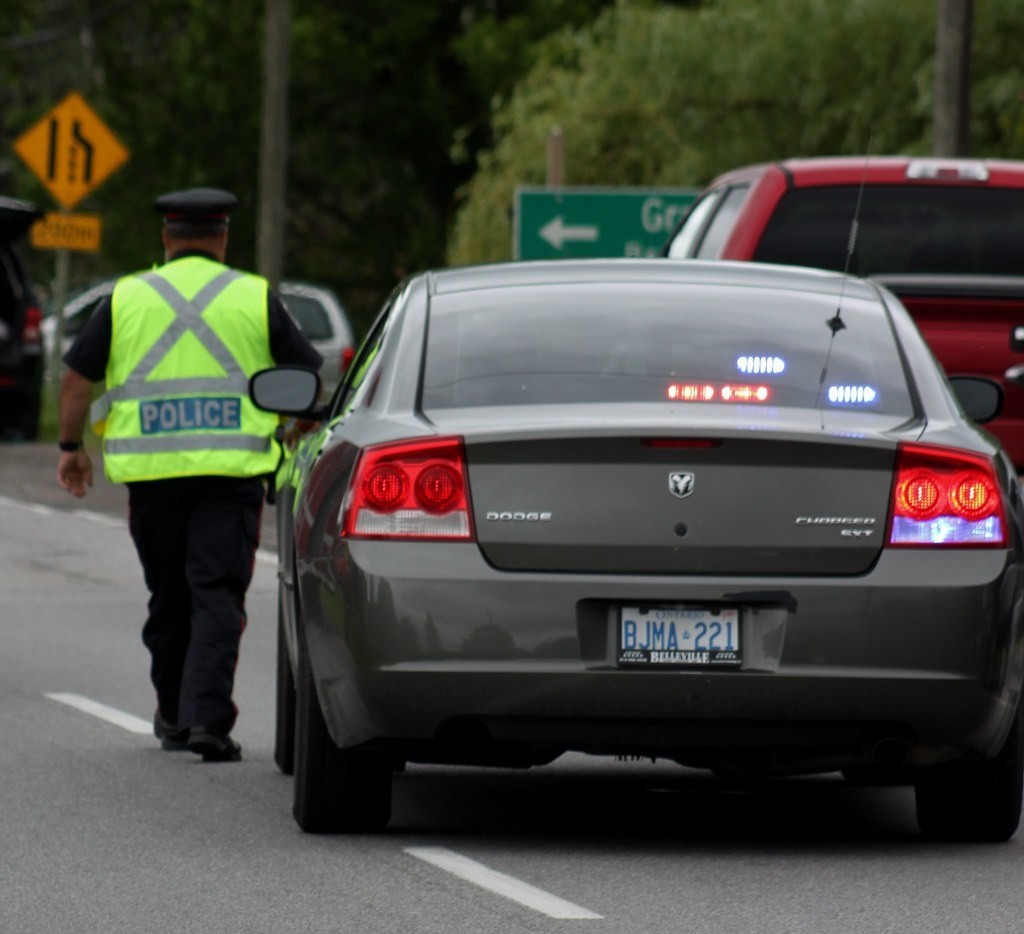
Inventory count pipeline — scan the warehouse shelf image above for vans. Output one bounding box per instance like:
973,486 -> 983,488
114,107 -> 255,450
659,157 -> 1022,488
40,272 -> 357,433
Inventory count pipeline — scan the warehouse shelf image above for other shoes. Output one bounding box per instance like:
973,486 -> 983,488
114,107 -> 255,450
186,728 -> 242,759
154,708 -> 186,749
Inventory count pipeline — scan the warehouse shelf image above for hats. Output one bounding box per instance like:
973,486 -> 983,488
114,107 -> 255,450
155,186 -> 237,225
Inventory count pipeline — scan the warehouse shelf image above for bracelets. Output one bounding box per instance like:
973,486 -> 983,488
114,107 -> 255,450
57,439 -> 86,453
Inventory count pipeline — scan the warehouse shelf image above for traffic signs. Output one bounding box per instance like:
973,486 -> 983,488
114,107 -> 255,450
514,191 -> 709,258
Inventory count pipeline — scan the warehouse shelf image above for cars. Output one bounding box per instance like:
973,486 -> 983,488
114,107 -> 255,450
246,260 -> 1020,842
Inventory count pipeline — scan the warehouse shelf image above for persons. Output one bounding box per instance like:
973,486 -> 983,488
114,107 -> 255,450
55,187 -> 324,760
